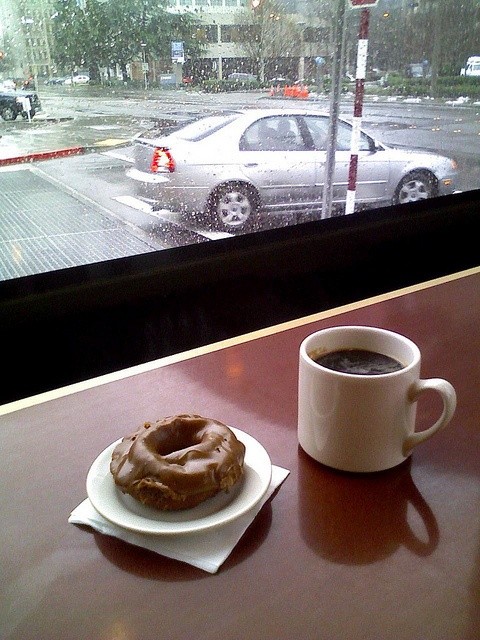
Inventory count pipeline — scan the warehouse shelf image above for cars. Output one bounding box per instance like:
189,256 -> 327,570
459,55 -> 480,77
126,108 -> 460,233
182,68 -> 312,101
65,75 -> 90,85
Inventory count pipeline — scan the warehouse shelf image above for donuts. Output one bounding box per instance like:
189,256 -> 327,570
110,413 -> 247,512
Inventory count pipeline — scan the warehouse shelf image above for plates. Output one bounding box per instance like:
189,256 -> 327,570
85,423 -> 272,536
92,499 -> 271,582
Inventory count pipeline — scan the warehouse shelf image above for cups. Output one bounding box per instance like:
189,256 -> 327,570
297,444 -> 440,565
297,323 -> 457,474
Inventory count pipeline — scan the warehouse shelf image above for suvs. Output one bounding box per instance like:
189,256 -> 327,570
0,86 -> 41,121
